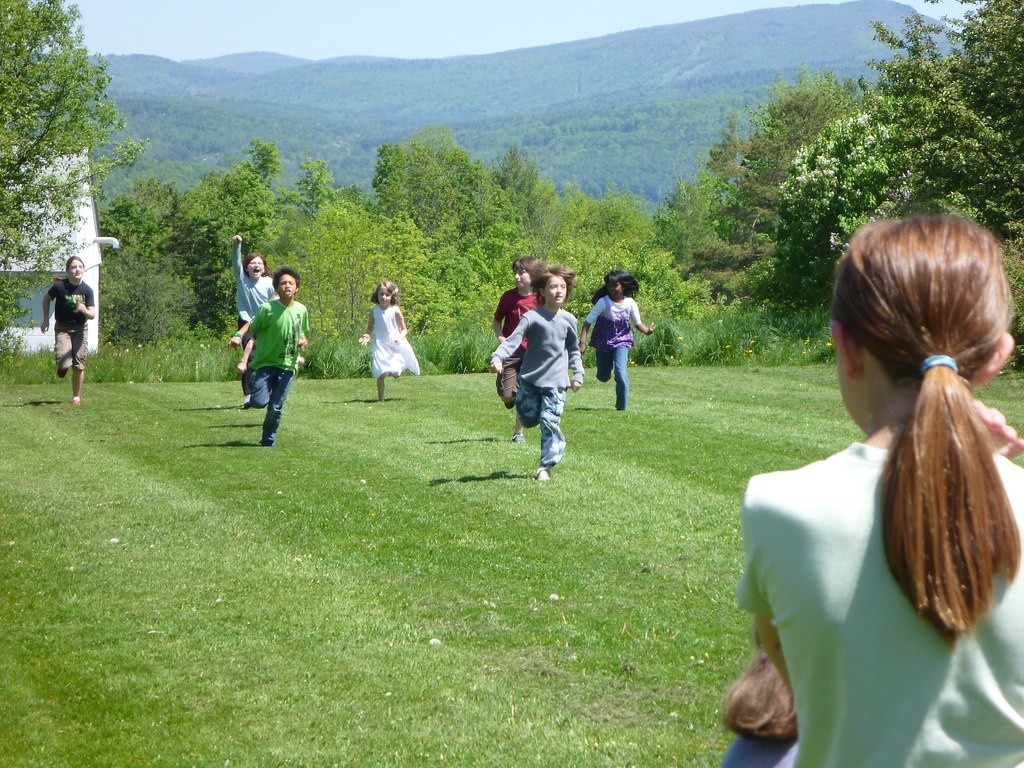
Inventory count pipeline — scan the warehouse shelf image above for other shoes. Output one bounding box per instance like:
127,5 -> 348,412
537,467 -> 550,481
512,432 -> 525,443
72,396 -> 80,405
243,393 -> 252,409
57,367 -> 68,377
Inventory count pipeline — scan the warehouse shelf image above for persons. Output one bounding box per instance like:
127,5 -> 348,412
491,265 -> 585,481
717,212 -> 1024,768
579,269 -> 655,411
232,234 -> 275,408
492,255 -> 544,444
227,267 -> 311,445
358,281 -> 419,402
40,256 -> 95,404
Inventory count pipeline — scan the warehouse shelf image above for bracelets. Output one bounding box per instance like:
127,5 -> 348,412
234,333 -> 244,339
